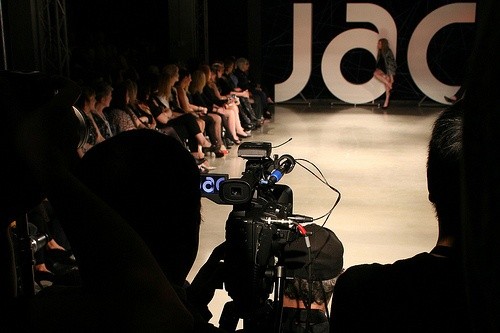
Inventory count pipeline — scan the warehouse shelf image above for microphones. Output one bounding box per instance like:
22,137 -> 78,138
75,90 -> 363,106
286,215 -> 313,225
271,136 -> 293,151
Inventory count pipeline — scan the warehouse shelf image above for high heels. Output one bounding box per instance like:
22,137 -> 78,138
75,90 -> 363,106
389,83 -> 395,92
381,101 -> 391,109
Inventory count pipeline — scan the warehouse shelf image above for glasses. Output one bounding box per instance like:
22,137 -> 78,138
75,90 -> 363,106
243,62 -> 250,65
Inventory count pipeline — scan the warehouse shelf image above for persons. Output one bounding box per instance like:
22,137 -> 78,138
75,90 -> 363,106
371,39 -> 398,109
326,105 -> 499,331
0,51 -> 334,333
445,35 -> 500,105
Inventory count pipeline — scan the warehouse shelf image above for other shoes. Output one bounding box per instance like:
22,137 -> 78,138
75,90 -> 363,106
195,97 -> 277,174
